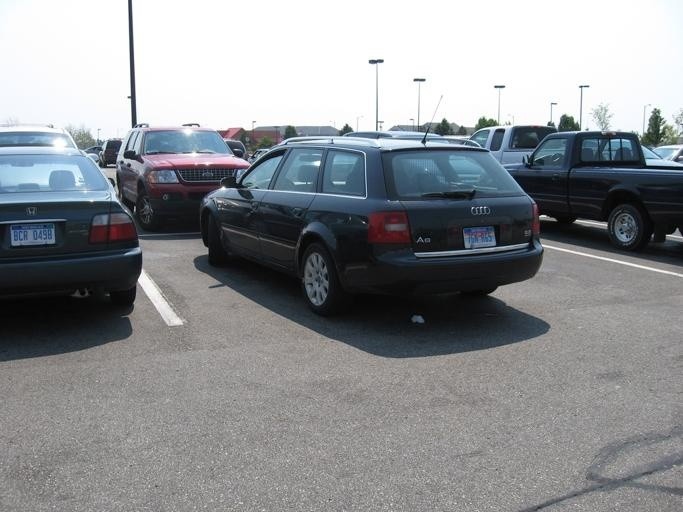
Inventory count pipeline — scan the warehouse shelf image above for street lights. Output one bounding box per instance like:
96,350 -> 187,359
367,58 -> 385,133
549,102 -> 558,122
408,119 -> 414,130
492,85 -> 506,125
577,85 -> 590,130
356,116 -> 364,132
412,78 -> 424,130
642,105 -> 651,134
